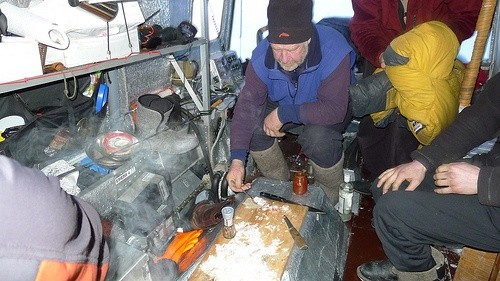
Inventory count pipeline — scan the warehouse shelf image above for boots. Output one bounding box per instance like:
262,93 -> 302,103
357,246 -> 445,281
180,189 -> 232,232
309,150 -> 346,207
134,92 -> 200,155
250,138 -> 292,182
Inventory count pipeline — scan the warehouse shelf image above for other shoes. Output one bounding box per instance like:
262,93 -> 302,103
152,258 -> 179,281
351,179 -> 373,196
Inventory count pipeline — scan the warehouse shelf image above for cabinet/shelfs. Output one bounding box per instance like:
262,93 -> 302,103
0,0 -> 215,170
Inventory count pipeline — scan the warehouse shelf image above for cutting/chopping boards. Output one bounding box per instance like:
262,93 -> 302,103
187,196 -> 307,280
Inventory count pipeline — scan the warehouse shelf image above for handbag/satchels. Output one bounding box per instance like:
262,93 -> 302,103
0,81 -> 93,169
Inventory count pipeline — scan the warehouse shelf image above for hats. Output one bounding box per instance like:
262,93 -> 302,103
266,0 -> 314,44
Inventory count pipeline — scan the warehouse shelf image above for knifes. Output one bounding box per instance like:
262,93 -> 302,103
260,192 -> 327,215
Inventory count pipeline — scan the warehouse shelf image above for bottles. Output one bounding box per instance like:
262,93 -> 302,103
293,172 -> 307,195
222,206 -> 236,240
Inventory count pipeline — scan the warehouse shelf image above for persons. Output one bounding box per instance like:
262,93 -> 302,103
0,155 -> 111,281
226,0 -> 356,207
348,0 -> 483,195
358,72 -> 500,281
